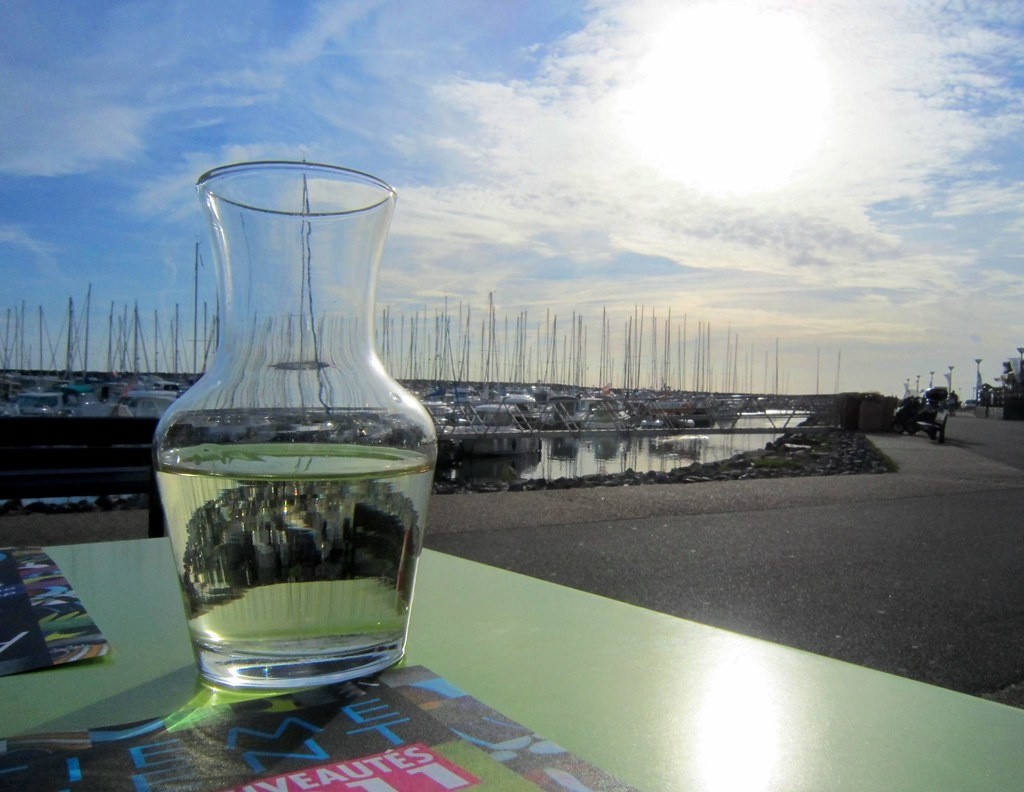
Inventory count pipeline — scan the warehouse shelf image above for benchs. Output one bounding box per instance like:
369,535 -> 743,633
915,412 -> 947,444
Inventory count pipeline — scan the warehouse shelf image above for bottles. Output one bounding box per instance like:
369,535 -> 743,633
156,155 -> 437,692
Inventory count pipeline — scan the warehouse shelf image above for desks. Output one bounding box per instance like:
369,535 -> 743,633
0,535 -> 1024,792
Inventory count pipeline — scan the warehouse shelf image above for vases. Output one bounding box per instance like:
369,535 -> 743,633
151,157 -> 440,694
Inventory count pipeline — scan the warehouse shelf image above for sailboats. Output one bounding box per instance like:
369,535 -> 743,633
0,286 -> 793,447
